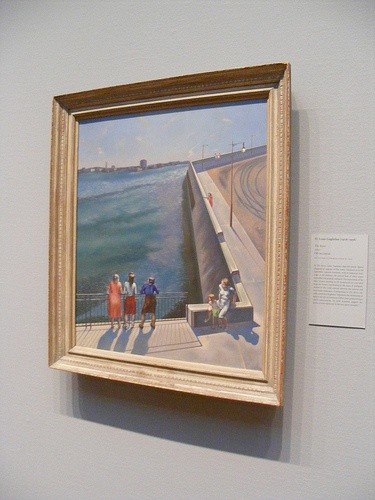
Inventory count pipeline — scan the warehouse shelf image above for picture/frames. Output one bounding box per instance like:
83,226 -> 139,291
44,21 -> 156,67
47,58 -> 294,407
